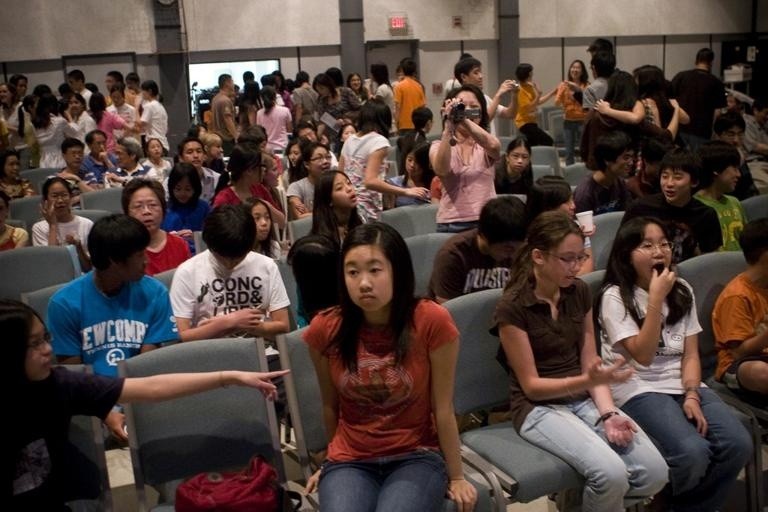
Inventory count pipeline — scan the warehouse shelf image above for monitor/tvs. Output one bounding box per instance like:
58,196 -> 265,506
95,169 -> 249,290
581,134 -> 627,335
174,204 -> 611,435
187,59 -> 281,118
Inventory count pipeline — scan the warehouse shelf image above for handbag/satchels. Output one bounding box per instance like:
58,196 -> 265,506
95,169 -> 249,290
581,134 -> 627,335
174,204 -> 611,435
171,455 -> 303,512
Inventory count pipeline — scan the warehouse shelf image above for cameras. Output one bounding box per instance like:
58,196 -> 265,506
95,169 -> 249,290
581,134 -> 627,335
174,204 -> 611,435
511,80 -> 519,90
451,102 -> 480,122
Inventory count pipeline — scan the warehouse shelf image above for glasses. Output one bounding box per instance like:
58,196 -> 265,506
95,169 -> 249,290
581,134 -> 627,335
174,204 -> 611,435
540,248 -> 590,268
47,193 -> 70,202
309,154 -> 332,162
28,333 -> 51,347
636,241 -> 675,255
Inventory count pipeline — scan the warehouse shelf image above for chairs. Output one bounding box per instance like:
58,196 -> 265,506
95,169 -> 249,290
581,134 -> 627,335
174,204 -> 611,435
0,78 -> 768,512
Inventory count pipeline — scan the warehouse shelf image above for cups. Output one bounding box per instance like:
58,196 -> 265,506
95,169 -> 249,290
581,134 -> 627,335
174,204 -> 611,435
575,209 -> 594,233
